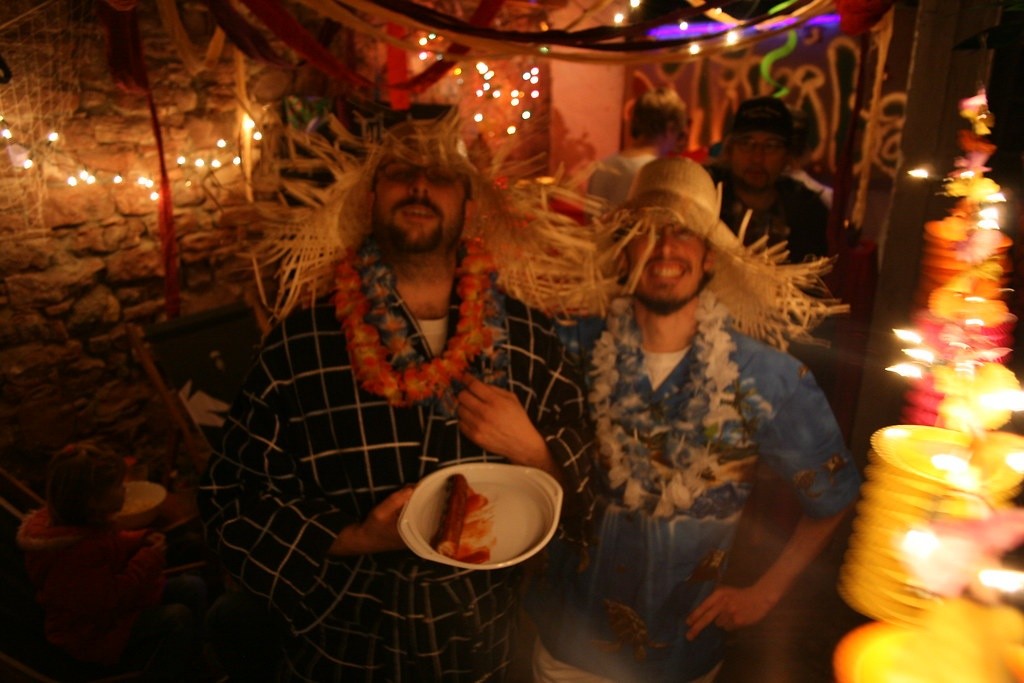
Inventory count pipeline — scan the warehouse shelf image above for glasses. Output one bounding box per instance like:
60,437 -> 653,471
733,136 -> 786,155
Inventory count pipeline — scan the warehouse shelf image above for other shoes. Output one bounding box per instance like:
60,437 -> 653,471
184,645 -> 230,683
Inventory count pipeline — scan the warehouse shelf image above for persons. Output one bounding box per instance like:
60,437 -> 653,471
786,112 -> 834,201
705,96 -> 831,299
588,89 -> 690,203
17,441 -> 228,683
532,195 -> 863,683
204,592 -> 291,683
201,154 -> 593,683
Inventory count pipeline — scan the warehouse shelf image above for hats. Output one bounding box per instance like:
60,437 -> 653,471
732,98 -> 792,147
496,158 -> 850,352
212,119 -> 604,319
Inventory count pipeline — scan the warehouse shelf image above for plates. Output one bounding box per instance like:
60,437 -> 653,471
832,212 -> 1024,683
396,462 -> 564,570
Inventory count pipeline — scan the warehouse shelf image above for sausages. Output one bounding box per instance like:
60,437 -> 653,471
433,474 -> 467,557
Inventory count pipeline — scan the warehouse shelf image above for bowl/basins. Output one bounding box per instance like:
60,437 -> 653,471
112,480 -> 168,529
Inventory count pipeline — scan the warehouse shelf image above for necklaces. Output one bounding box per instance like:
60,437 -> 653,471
336,236 -> 497,405
591,291 -> 739,517
